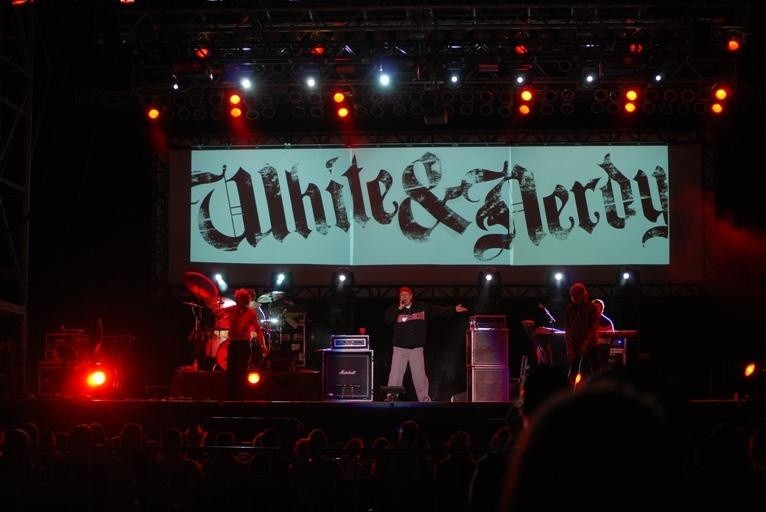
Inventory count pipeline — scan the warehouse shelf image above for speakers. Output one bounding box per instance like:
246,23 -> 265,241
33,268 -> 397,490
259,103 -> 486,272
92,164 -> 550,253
466,328 -> 509,367
467,367 -> 509,402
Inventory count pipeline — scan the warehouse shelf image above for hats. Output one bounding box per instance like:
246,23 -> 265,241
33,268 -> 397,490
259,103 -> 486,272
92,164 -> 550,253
400,287 -> 412,293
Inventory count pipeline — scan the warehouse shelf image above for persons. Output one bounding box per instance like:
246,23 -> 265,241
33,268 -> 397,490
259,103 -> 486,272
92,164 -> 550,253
215,291 -> 270,398
385,287 -> 469,404
590,296 -> 615,371
566,280 -> 602,392
247,289 -> 266,321
1,385 -> 765,510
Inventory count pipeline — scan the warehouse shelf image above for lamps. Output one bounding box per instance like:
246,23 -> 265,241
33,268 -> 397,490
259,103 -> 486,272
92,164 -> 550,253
205,272 -> 229,281
477,268 -> 501,282
270,271 -> 293,286
616,268 -> 639,282
331,266 -> 354,287
547,268 -> 571,283
378,385 -> 406,406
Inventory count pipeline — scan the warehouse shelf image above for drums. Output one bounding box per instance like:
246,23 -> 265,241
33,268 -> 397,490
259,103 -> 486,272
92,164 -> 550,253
216,339 -> 231,373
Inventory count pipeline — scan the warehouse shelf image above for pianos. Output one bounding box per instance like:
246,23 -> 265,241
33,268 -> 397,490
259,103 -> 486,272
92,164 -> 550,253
553,330 -> 640,338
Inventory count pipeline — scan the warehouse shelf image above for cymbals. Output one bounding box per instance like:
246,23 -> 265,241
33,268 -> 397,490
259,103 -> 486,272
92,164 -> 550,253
184,301 -> 205,309
257,290 -> 286,304
217,296 -> 237,308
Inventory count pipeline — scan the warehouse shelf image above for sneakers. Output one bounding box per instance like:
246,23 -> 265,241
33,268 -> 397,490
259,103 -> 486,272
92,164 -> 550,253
384,396 -> 398,402
